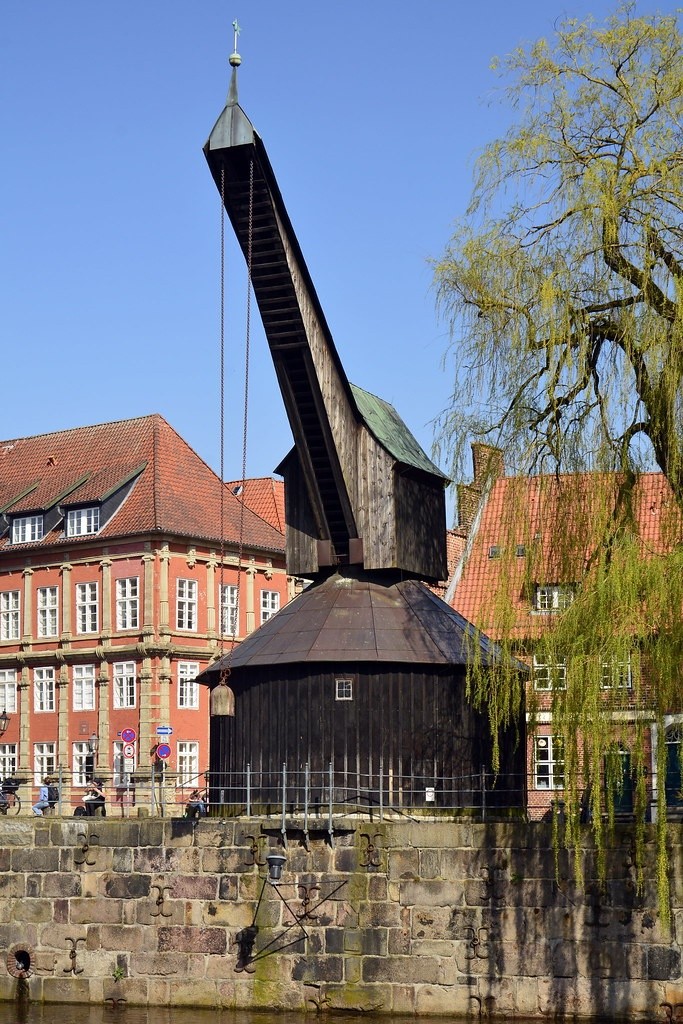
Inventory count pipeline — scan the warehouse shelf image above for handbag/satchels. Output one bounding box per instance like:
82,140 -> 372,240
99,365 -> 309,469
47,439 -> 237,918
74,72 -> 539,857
82,795 -> 97,803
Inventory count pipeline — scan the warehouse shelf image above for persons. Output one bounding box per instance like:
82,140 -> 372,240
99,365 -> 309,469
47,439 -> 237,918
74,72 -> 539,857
31,777 -> 51,818
182,790 -> 204,819
83,778 -> 106,815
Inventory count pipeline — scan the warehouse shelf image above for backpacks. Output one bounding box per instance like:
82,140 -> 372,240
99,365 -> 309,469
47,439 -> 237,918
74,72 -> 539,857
46,785 -> 59,804
74,806 -> 86,816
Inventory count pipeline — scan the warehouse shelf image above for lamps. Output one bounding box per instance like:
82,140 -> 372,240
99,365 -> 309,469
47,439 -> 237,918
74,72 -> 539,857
88,731 -> 101,755
266,854 -> 286,878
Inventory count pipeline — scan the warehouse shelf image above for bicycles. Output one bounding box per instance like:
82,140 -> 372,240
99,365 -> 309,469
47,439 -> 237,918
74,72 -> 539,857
0,789 -> 20,820
